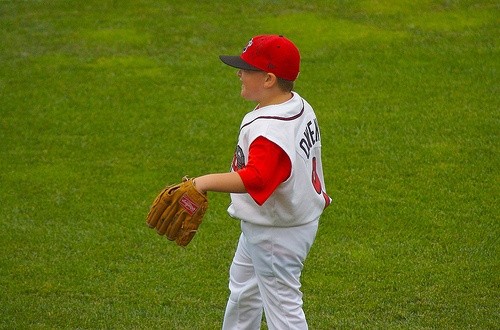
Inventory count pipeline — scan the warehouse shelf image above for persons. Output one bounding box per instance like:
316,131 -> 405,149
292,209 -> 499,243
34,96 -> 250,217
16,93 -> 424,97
145,34 -> 333,330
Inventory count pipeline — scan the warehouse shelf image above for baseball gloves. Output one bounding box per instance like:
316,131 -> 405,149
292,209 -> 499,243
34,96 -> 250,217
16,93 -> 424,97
145,175 -> 208,246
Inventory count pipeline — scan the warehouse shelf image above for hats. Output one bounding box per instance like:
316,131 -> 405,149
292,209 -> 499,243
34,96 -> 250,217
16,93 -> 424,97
218,33 -> 300,81
